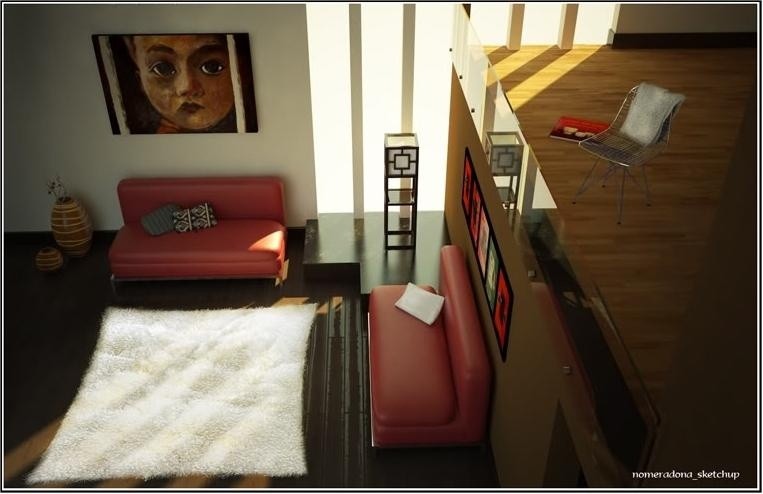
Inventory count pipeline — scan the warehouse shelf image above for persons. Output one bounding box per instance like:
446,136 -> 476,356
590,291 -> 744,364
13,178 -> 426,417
122,35 -> 234,134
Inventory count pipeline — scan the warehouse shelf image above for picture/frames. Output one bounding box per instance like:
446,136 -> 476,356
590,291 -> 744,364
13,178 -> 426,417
458,139 -> 516,363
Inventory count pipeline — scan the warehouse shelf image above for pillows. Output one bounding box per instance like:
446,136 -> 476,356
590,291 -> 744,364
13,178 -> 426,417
395,279 -> 446,327
139,201 -> 181,236
169,201 -> 218,233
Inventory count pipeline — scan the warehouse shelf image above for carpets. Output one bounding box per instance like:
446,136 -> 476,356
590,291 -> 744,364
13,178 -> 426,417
24,298 -> 321,487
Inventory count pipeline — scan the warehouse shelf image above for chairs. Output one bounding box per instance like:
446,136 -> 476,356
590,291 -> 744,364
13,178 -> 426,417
568,78 -> 685,227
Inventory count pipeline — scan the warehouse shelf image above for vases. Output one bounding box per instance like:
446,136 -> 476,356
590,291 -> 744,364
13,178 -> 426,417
32,247 -> 65,277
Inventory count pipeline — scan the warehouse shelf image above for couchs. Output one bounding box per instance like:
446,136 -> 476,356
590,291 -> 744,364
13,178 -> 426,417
106,173 -> 289,292
363,241 -> 495,454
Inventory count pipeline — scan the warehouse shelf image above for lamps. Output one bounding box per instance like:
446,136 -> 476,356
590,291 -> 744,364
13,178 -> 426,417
381,129 -> 420,256
482,130 -> 525,235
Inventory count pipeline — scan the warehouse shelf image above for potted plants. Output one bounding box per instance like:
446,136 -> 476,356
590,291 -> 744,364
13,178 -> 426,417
45,173 -> 97,259
